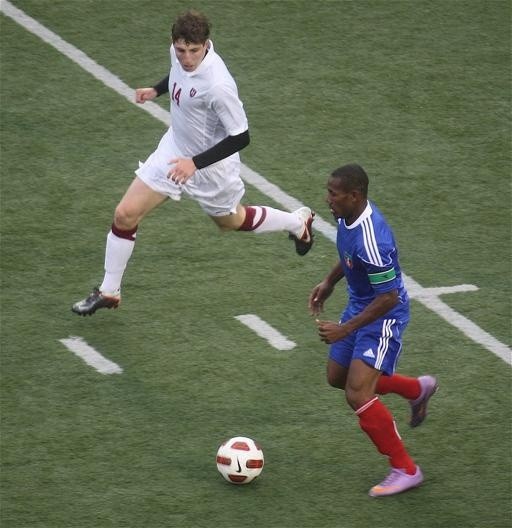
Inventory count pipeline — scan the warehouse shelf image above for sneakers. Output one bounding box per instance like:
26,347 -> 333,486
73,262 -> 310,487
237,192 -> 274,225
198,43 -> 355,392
367,464 -> 424,498
407,375 -> 438,428
69,284 -> 122,318
287,206 -> 315,256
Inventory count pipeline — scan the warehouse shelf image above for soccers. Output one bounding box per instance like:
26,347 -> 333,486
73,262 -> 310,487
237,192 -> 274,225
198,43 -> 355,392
216,437 -> 265,484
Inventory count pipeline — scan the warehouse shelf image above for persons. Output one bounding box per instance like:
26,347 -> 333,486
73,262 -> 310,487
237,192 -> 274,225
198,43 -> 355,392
68,11 -> 317,319
308,160 -> 439,498
